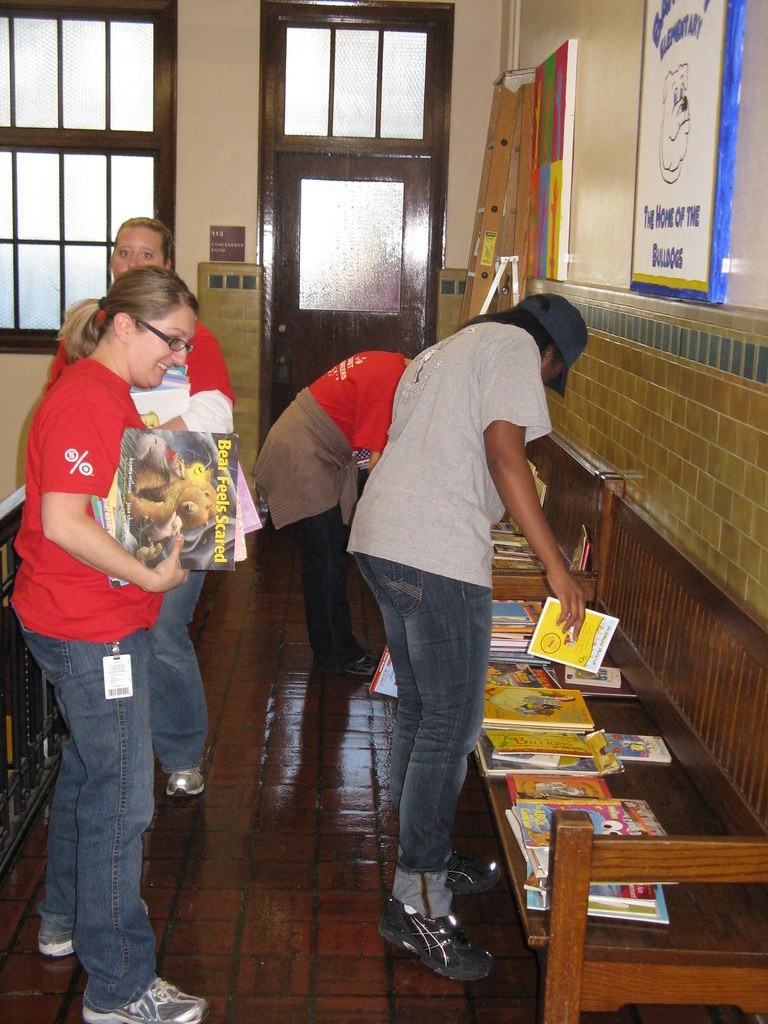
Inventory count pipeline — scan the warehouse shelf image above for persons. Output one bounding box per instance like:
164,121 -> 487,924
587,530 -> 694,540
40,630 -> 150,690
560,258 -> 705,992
44,215 -> 233,795
252,351 -> 415,676
10,267 -> 212,1024
348,293 -> 588,984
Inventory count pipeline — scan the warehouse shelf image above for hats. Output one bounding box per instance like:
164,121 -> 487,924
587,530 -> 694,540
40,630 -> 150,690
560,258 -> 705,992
522,294 -> 589,396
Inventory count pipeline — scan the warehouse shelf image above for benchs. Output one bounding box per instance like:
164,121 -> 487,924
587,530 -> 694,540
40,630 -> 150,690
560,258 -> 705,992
488,433 -> 626,572
471,477 -> 768,1024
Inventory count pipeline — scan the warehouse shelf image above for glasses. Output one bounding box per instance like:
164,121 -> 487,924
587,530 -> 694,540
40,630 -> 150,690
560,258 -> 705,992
125,312 -> 195,355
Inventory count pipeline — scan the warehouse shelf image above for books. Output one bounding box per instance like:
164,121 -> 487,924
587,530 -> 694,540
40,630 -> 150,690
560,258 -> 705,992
507,457 -> 547,537
506,774 -> 681,925
88,384 -> 262,590
369,644 -> 399,698
527,595 -> 620,674
491,522 -> 590,576
475,686 -> 673,777
485,600 -> 638,698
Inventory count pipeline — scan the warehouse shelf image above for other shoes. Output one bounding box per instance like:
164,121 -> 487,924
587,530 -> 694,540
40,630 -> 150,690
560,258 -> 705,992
316,654 -> 379,676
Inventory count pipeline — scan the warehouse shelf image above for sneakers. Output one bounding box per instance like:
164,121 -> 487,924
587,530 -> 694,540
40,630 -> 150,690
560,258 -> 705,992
82,978 -> 209,1024
378,896 -> 494,981
38,891 -> 148,955
165,766 -> 204,797
445,849 -> 501,895
